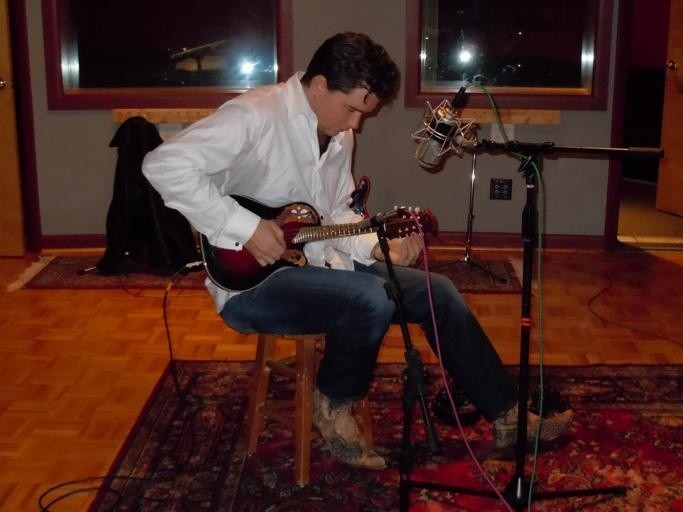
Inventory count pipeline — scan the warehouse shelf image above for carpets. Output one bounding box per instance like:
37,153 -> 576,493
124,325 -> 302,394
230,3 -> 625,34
7,249 -> 524,296
86,358 -> 683,512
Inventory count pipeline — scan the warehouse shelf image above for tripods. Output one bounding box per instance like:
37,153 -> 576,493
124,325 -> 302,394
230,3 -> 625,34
406,205 -> 629,512
429,154 -> 509,285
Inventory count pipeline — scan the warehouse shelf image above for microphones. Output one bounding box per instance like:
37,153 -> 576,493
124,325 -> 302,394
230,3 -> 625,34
413,85 -> 468,174
169,36 -> 235,65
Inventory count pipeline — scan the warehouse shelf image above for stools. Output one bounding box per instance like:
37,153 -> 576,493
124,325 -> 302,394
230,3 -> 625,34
243,334 -> 373,488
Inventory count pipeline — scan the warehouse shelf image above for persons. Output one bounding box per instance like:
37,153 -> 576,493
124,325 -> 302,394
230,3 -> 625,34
140,30 -> 576,471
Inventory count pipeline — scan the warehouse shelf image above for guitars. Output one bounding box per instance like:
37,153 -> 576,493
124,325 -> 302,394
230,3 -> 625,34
199,177 -> 438,291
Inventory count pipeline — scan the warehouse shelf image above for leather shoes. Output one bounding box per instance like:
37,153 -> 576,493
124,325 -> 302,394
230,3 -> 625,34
313,388 -> 386,469
493,407 -> 573,450
